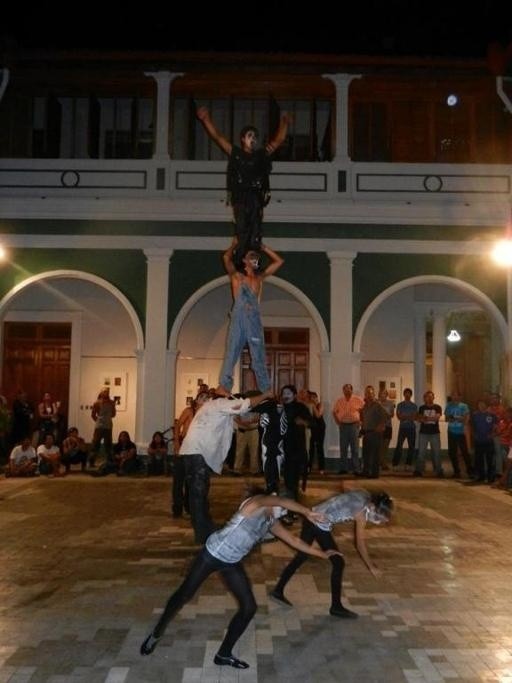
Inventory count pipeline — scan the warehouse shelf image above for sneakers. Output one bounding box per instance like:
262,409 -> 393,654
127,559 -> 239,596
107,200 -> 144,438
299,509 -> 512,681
214,653 -> 249,669
330,606 -> 358,619
141,632 -> 162,656
269,591 -> 294,609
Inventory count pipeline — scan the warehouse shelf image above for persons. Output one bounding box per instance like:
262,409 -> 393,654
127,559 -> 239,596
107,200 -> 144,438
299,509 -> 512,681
171,383 -> 327,543
196,102 -> 293,272
333,383 -> 511,490
216,233 -> 285,398
3,383 -> 169,478
277,480 -> 394,614
139,484 -> 345,669
